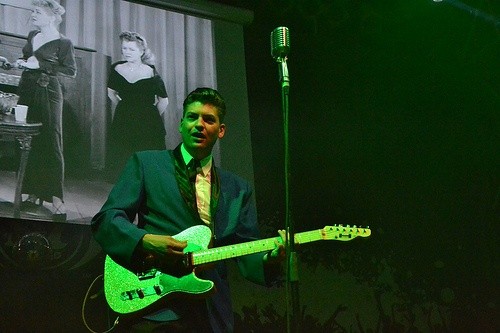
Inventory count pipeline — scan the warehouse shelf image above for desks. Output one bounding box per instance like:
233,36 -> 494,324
0,112 -> 44,220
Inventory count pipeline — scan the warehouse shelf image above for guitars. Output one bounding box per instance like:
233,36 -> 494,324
102,224 -> 371,315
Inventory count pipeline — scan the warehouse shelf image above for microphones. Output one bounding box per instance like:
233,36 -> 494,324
270,26 -> 291,61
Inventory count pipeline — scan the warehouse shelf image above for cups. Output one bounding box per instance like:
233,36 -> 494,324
15,105 -> 28,122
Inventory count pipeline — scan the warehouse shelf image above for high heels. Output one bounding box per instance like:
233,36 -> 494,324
21,196 -> 42,208
53,205 -> 66,222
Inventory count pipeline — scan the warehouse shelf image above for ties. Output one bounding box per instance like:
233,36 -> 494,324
189,158 -> 198,216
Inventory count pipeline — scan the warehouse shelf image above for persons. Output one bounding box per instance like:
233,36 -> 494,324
89,86 -> 299,333
16,0 -> 77,206
106,32 -> 168,184
